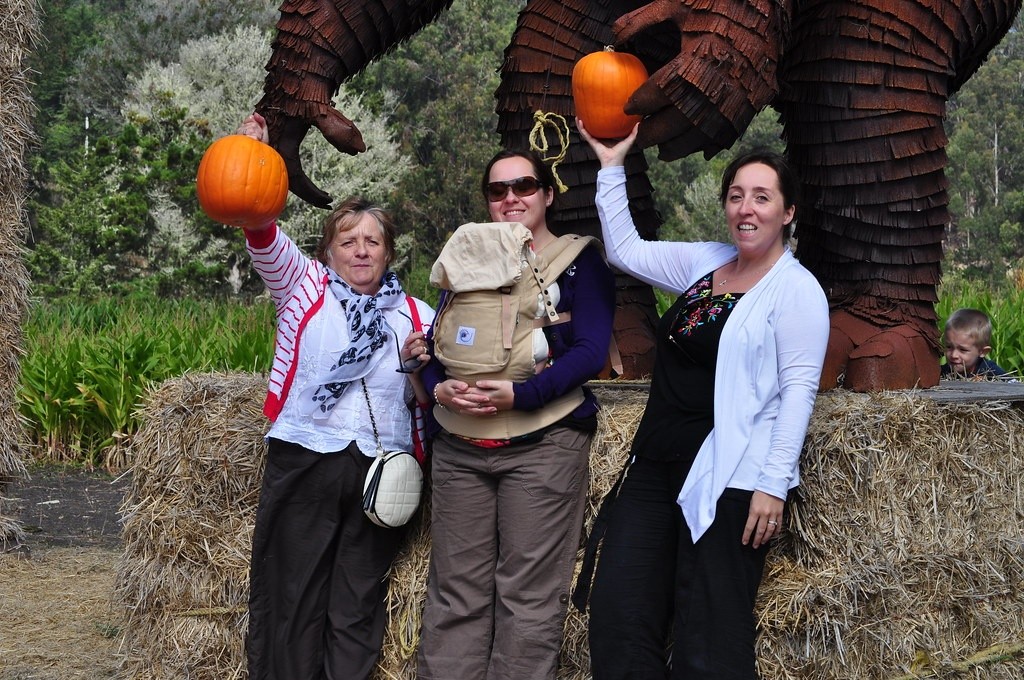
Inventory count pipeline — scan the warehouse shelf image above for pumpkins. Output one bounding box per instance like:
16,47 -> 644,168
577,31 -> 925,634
197,135 -> 289,227
572,45 -> 648,139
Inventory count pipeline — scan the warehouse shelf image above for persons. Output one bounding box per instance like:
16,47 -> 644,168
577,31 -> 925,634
939,308 -> 1014,382
236,112 -> 832,680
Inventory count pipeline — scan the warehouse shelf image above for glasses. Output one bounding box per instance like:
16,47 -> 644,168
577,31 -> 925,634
485,176 -> 545,202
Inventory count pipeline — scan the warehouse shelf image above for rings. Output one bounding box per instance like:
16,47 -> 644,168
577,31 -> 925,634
768,520 -> 778,526
477,402 -> 482,409
243,128 -> 247,135
423,346 -> 429,354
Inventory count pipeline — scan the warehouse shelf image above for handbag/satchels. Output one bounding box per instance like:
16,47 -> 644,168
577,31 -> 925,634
363,448 -> 426,529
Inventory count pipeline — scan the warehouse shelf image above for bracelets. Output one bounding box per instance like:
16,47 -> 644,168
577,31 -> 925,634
433,382 -> 446,409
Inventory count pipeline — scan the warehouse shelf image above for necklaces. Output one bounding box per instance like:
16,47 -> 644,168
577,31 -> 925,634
717,259 -> 775,288
